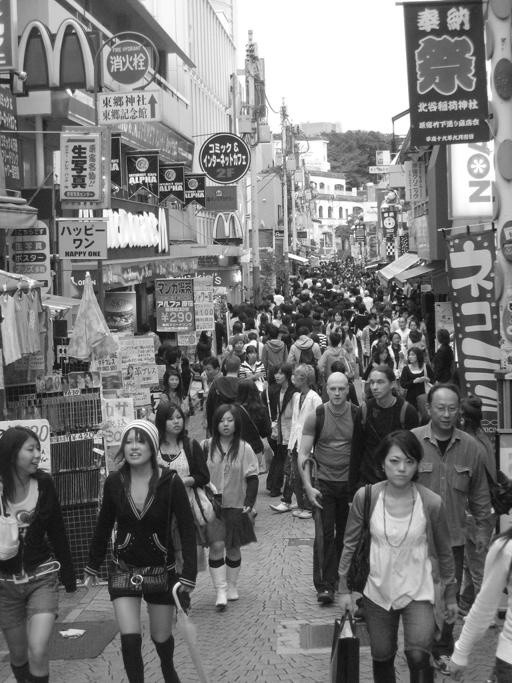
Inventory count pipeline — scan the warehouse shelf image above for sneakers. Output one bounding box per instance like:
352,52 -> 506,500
291,509 -> 303,516
433,656 -> 454,675
299,510 -> 312,518
270,503 -> 292,512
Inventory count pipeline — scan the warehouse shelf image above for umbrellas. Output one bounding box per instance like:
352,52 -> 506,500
170,582 -> 207,683
67,271 -> 120,366
302,458 -> 325,579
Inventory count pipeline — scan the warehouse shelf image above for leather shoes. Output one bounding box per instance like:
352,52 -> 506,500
317,589 -> 334,602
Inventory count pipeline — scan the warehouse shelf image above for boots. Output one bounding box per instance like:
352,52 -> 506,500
225,556 -> 241,601
208,559 -> 227,607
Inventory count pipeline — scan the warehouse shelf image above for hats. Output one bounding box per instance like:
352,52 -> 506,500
119,418 -> 160,456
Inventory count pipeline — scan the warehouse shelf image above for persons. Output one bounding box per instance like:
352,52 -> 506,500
163,347 -> 185,398
201,356 -> 224,440
154,403 -> 210,609
195,313 -> 323,383
228,262 -> 422,313
0,426 -> 77,682
266,383 -> 286,498
361,368 -> 417,484
410,384 -> 496,675
201,405 -> 258,608
205,355 -> 273,454
269,363 -> 296,496
319,313 -> 454,405
159,370 -> 190,416
337,430 -> 460,682
83,420 -> 197,683
460,393 -> 496,627
269,365 -> 324,519
448,526 -> 511,682
297,371 -> 362,602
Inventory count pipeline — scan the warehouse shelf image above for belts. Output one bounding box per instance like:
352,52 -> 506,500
0,557 -> 61,585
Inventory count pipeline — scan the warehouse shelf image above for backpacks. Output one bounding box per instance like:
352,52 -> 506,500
295,343 -> 317,370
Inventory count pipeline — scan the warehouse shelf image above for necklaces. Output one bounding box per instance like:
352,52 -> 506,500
382,487 -> 415,548
164,444 -> 180,459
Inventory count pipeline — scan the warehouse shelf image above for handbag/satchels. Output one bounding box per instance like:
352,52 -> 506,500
423,362 -> 438,393
271,420 -> 279,440
261,437 -> 275,466
331,610 -> 359,683
189,486 -> 216,525
347,485 -> 373,593
165,563 -> 190,617
205,485 -> 222,518
191,351 -> 202,372
484,465 -> 512,514
0,498 -> 19,559
340,355 -> 355,379
